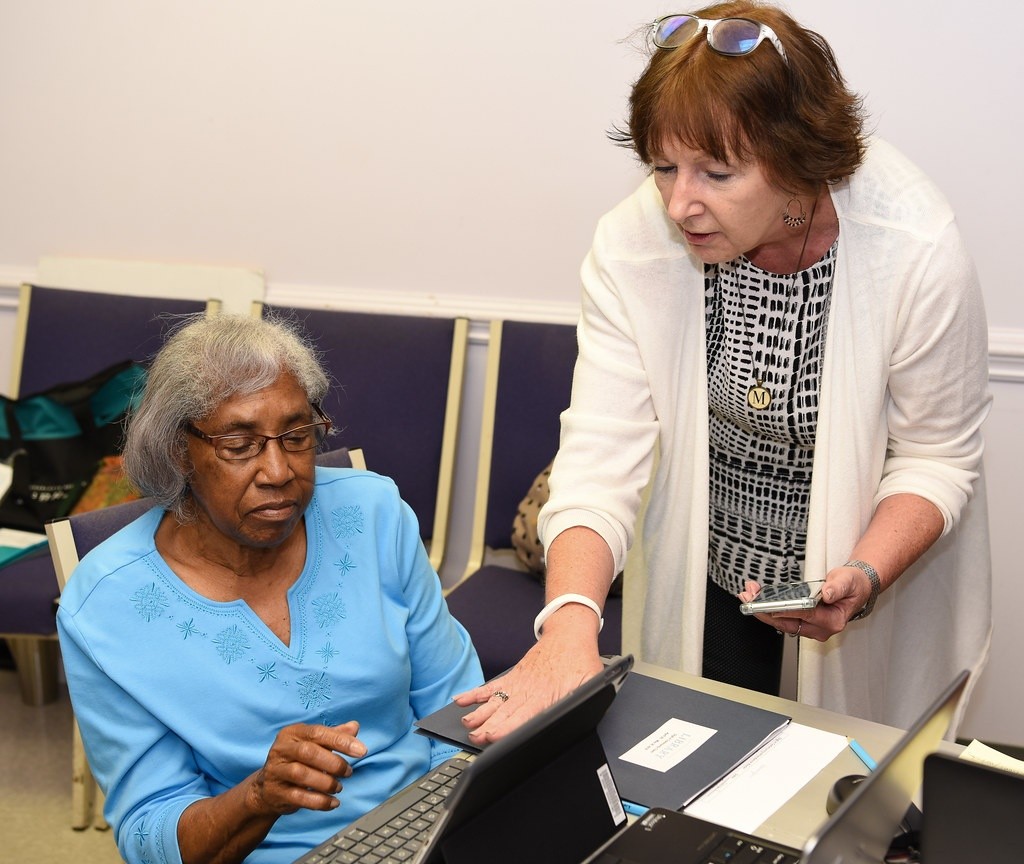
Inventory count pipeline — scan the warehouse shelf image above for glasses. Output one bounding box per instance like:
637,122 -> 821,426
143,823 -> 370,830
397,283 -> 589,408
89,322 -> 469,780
652,13 -> 790,67
183,402 -> 332,461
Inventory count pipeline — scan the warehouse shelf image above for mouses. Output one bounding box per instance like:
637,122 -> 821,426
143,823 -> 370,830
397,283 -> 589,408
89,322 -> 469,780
826,774 -> 924,859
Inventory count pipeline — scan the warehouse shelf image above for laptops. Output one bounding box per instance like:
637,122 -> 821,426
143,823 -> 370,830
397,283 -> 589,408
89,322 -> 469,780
585,668 -> 971,864
291,655 -> 637,864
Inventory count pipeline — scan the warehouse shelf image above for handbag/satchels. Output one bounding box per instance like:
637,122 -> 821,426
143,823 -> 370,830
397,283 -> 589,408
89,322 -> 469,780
0,359 -> 151,537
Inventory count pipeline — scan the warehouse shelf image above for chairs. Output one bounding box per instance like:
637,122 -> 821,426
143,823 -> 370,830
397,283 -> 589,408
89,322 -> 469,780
0,282 -> 663,832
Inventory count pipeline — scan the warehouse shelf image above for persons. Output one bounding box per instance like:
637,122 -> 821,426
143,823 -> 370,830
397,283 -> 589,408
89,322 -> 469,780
454,0 -> 992,744
54,308 -> 485,864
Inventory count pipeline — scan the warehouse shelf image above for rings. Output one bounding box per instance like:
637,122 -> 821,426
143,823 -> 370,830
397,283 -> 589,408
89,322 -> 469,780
788,622 -> 801,637
777,630 -> 785,635
494,690 -> 508,701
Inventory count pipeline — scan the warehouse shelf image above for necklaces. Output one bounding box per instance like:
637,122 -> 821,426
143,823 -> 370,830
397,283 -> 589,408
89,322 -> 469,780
732,186 -> 821,409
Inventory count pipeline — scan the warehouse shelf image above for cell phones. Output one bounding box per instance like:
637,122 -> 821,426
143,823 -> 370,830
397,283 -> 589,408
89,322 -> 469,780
740,580 -> 825,616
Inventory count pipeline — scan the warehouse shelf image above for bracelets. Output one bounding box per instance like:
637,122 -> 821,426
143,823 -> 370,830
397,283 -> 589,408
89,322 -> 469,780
843,559 -> 880,620
533,593 -> 606,640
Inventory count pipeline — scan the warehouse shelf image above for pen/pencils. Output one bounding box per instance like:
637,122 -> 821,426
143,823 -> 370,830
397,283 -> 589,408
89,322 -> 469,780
845,735 -> 878,772
621,800 -> 650,816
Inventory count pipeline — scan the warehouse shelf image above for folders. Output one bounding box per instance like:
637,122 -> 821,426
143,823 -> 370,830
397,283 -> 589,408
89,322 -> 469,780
413,662 -> 792,812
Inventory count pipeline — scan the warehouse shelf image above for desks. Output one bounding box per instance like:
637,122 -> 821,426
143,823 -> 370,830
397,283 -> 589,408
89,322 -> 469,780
457,654 -> 1024,864
